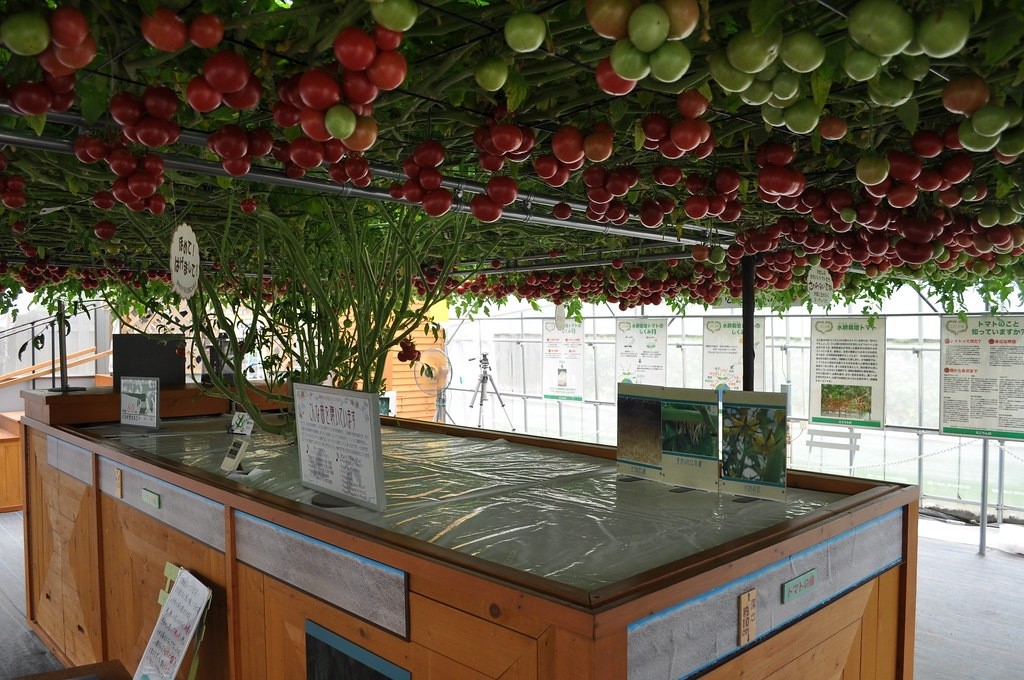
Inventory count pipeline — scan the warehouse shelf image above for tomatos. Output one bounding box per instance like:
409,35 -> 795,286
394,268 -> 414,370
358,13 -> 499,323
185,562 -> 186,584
0,0 -> 1024,362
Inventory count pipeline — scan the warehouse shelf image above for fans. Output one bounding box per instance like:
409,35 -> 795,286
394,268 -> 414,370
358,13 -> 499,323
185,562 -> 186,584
414,348 -> 455,424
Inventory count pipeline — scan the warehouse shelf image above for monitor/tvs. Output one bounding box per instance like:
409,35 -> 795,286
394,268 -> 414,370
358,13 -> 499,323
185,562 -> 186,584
113,333 -> 186,393
